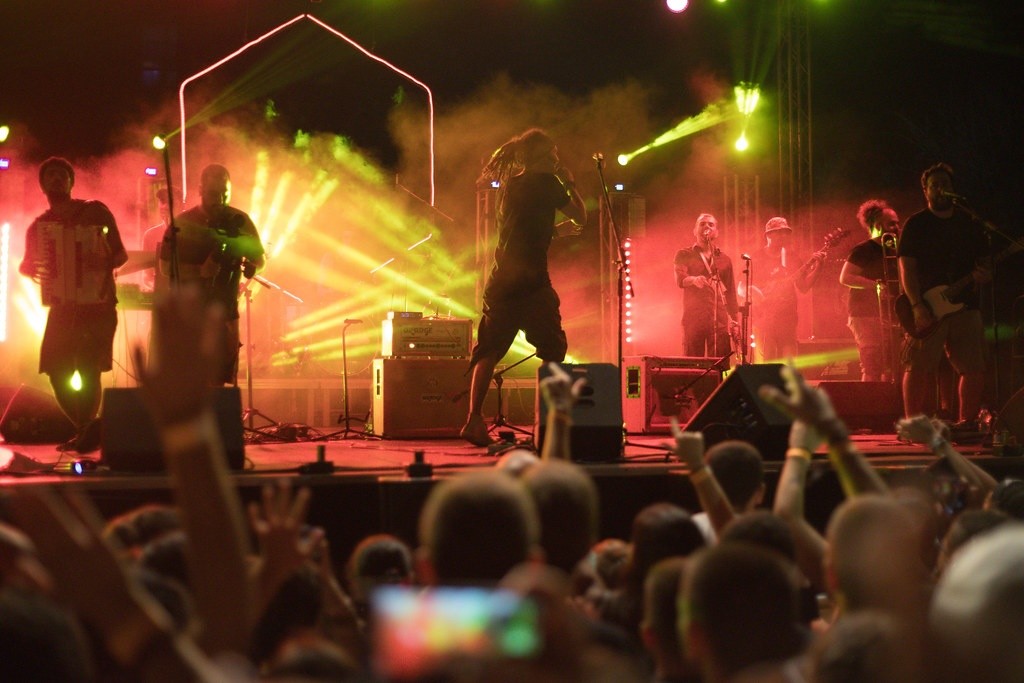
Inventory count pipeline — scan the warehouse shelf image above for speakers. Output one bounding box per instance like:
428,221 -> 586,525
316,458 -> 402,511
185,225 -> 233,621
1,383 -> 77,444
811,378 -> 904,432
981,388 -> 1024,452
99,385 -> 246,470
681,362 -> 807,461
532,363 -> 621,461
368,359 -> 474,439
617,354 -> 729,432
481,377 -> 535,426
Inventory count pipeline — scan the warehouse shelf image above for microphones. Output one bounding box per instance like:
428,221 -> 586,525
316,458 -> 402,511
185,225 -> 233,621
592,153 -> 604,161
702,229 -> 712,236
939,190 -> 967,202
739,253 -> 751,261
344,319 -> 365,324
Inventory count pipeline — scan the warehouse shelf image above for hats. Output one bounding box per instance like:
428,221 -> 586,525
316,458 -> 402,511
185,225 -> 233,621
763,217 -> 792,239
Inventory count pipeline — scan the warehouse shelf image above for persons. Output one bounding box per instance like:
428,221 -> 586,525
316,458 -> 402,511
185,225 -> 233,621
158,163 -> 266,387
675,212 -> 739,357
18,155 -> 127,450
839,198 -> 899,381
458,127 -> 587,447
899,163 -> 995,421
749,216 -> 826,360
143,186 -> 186,261
0,361 -> 1024,683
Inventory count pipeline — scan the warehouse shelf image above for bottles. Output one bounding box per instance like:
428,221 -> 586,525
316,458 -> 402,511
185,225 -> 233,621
976,402 -> 992,433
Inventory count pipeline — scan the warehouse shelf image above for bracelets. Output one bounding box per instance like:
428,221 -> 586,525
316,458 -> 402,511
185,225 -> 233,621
193,263 -> 201,278
784,446 -> 815,465
563,181 -> 575,189
930,434 -> 945,451
689,463 -> 713,482
551,225 -> 558,240
159,405 -> 219,452
816,416 -> 847,443
910,301 -> 923,309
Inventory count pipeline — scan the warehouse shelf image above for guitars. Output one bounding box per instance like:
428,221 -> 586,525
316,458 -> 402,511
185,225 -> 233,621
740,227 -> 852,313
894,236 -> 1024,341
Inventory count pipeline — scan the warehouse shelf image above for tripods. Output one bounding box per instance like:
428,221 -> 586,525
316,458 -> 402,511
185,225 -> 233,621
451,353 -> 536,435
310,323 -> 382,439
235,274 -> 284,436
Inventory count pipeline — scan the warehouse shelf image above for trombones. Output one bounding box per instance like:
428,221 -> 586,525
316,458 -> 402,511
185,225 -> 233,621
879,232 -> 914,380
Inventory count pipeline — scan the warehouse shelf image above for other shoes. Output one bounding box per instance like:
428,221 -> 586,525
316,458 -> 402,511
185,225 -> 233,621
53,430 -> 87,451
460,414 -> 493,446
